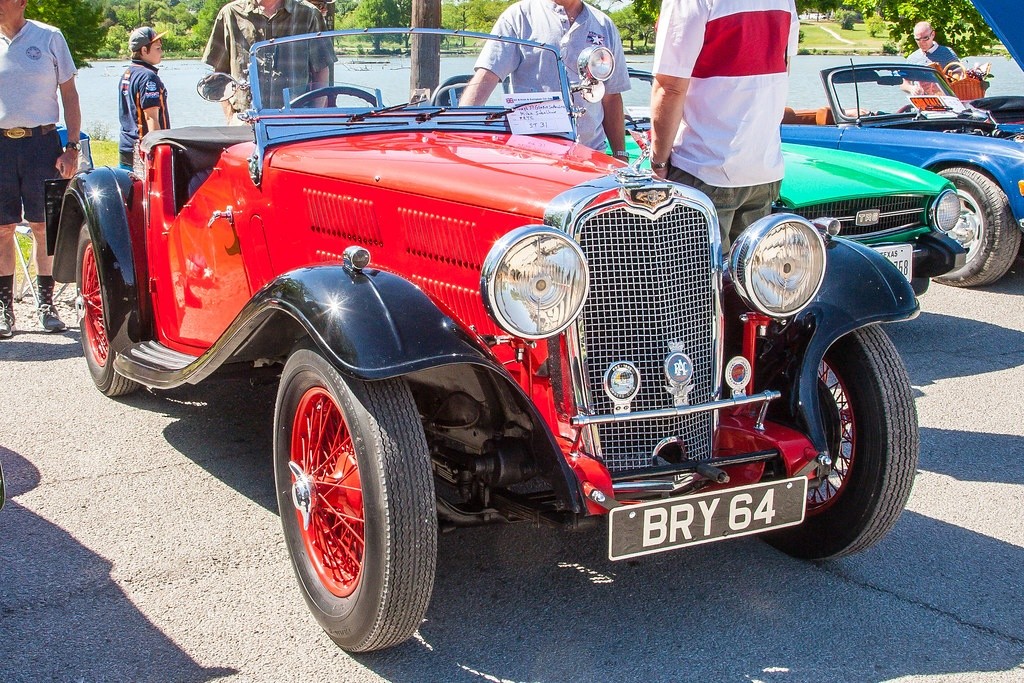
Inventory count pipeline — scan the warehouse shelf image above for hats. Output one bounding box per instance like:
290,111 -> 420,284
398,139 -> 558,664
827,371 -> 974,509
129,26 -> 168,51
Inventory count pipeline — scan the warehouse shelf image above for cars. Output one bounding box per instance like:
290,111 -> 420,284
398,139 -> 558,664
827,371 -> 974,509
626,1 -> 1024,288
429,64 -> 968,299
52,30 -> 922,655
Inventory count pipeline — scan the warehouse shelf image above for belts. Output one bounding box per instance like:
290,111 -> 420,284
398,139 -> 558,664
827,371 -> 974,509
1,125 -> 57,139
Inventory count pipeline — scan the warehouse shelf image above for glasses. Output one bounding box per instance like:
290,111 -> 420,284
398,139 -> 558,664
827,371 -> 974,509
913,30 -> 933,41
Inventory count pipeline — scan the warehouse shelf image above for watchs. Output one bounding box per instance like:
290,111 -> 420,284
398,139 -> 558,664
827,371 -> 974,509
66,142 -> 82,152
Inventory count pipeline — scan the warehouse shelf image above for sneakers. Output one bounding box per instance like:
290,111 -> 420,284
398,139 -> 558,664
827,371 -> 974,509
32,304 -> 67,333
0,300 -> 16,337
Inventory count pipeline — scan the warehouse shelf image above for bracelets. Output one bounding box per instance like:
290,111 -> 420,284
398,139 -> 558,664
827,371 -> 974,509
613,151 -> 629,160
649,156 -> 669,168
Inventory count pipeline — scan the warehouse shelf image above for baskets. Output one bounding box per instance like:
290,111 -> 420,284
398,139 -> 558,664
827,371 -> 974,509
942,62 -> 986,100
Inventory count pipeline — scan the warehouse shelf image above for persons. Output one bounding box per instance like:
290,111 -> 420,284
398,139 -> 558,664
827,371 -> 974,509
900,21 -> 964,96
649,0 -> 800,257
0,0 -> 82,339
118,26 -> 171,172
201,0 -> 338,126
458,0 -> 632,164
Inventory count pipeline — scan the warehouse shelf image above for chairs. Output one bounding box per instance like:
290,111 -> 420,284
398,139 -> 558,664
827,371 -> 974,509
13,125 -> 94,308
816,106 -> 846,126
